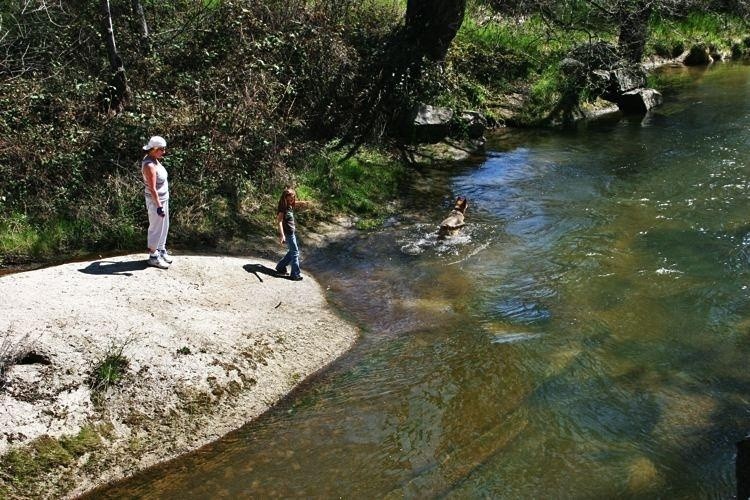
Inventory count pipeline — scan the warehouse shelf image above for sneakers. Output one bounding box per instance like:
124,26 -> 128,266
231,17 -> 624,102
159,249 -> 173,264
290,275 -> 303,281
276,270 -> 289,275
147,254 -> 171,269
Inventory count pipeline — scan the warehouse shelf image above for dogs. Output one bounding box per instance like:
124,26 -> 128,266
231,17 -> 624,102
438,196 -> 469,235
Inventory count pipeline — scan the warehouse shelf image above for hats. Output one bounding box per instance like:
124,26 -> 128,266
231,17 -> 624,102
142,135 -> 167,151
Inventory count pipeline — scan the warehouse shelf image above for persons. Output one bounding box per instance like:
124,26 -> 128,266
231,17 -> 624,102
275,189 -> 303,281
141,136 -> 172,269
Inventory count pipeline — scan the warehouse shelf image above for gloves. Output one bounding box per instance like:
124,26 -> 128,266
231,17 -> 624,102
157,207 -> 165,218
